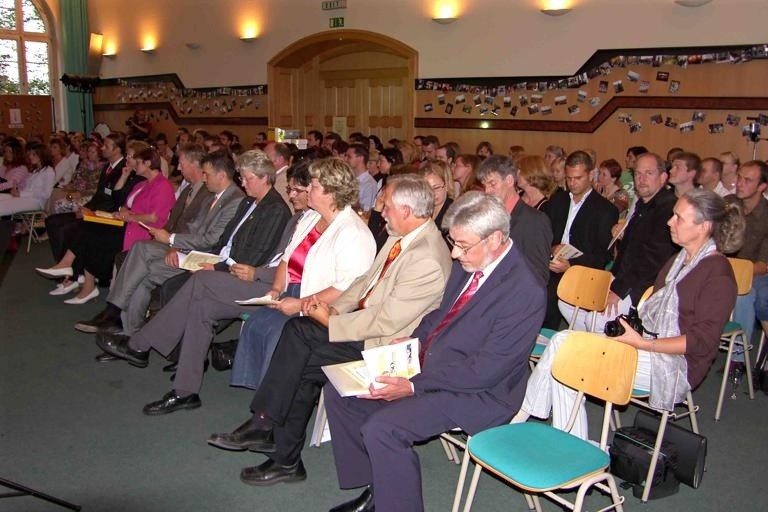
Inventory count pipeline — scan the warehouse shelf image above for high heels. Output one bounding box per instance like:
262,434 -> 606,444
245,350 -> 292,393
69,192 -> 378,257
35,265 -> 99,306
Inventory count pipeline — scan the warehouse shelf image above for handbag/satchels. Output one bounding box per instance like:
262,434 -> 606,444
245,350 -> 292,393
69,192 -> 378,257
210,338 -> 240,371
609,424 -> 682,500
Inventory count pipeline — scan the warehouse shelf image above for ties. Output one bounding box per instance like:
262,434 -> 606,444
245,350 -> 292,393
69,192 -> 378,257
358,239 -> 404,310
106,166 -> 113,176
418,270 -> 484,369
209,197 -> 219,213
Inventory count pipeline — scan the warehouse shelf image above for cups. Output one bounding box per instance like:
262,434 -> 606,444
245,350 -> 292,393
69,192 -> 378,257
727,371 -> 742,400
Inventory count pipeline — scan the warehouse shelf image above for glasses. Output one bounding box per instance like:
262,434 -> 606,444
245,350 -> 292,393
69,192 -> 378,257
286,186 -> 306,194
446,234 -> 484,256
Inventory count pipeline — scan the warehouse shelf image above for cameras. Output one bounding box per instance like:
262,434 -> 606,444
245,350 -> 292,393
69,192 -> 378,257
605,306 -> 644,337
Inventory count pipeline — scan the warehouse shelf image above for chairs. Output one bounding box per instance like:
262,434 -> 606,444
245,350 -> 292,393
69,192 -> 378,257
714,258 -> 755,421
438,427 -> 536,510
612,287 -> 700,502
463,329 -> 639,510
529,266 -> 614,371
18,196 -> 49,252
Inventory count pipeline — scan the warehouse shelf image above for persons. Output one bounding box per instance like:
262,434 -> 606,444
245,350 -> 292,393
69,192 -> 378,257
0,110 -> 768,381
95,162 -> 310,415
510,191 -> 748,441
206,174 -> 452,485
323,191 -> 547,512
229,159 -> 378,389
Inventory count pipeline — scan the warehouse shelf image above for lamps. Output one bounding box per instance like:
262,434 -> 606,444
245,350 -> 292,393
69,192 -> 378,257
433,0 -> 459,26
540,2 -> 572,17
240,22 -> 257,42
58,73 -> 100,114
140,36 -> 156,55
102,44 -> 116,58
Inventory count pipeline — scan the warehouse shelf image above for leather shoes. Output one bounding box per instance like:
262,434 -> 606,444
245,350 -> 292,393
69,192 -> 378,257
240,450 -> 307,487
143,388 -> 203,417
73,314 -> 150,370
326,488 -> 375,512
207,415 -> 277,453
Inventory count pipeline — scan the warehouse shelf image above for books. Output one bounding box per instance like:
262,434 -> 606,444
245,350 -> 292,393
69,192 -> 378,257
358,339 -> 421,388
549,244 -> 583,264
175,251 -> 222,271
